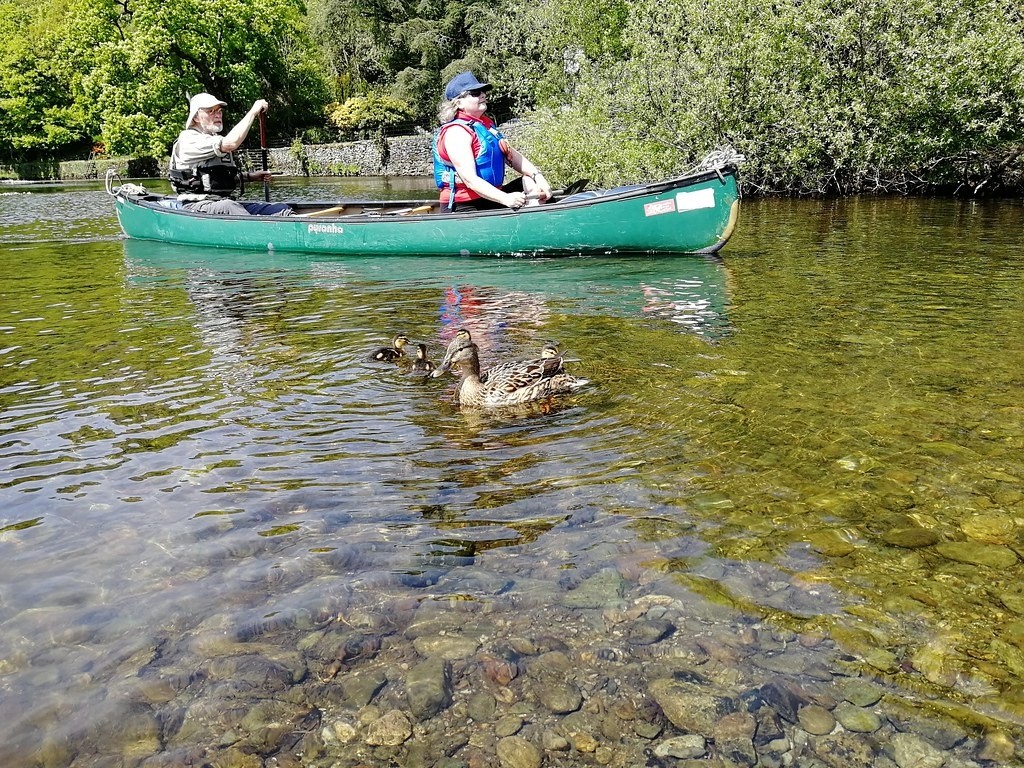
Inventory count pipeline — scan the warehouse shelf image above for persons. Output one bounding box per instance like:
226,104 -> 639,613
168,92 -> 297,216
432,71 -> 554,213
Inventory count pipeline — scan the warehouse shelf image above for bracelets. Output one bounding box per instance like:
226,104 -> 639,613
247,172 -> 253,180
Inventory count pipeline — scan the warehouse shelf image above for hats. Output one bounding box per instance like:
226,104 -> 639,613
186,93 -> 227,130
446,72 -> 494,101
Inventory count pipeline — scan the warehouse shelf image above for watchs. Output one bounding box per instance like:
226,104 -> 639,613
531,171 -> 542,184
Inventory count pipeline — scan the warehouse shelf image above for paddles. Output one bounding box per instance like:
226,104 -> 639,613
258,108 -> 271,204
512,177 -> 590,212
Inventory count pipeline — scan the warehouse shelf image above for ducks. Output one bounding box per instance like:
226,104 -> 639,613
369,333 -> 410,361
430,329 -> 591,407
395,343 -> 440,374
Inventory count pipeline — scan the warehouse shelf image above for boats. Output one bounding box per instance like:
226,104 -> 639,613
103,163 -> 744,258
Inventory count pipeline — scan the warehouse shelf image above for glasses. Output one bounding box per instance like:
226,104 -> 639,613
466,88 -> 487,97
199,108 -> 223,115
